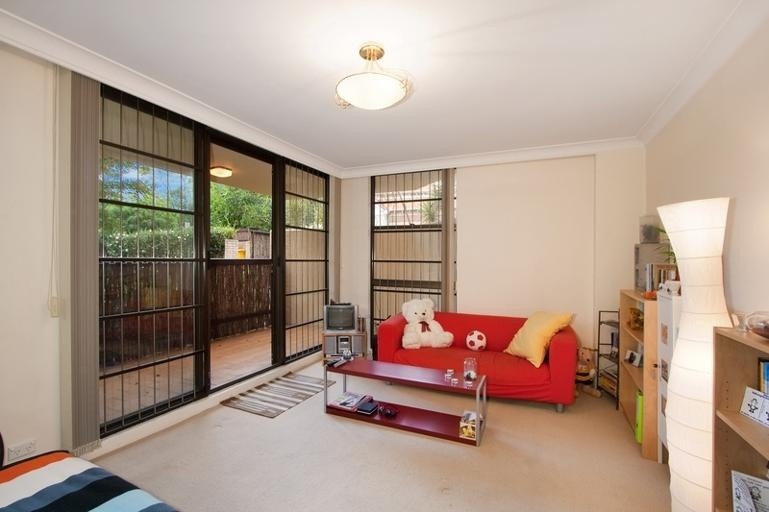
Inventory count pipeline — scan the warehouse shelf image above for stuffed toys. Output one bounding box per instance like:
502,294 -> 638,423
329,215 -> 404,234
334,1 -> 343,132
400,298 -> 455,350
574,347 -> 602,397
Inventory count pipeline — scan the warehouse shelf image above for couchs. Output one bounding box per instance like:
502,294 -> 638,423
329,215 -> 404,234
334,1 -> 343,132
374,312 -> 580,413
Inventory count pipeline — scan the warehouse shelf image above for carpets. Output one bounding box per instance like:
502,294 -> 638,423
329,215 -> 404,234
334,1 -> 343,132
221,366 -> 336,420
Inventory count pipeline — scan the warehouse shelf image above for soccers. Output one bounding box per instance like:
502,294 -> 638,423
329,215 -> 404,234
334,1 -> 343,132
466,330 -> 487,351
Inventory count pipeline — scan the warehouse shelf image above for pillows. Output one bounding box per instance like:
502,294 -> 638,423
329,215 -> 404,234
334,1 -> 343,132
502,309 -> 576,368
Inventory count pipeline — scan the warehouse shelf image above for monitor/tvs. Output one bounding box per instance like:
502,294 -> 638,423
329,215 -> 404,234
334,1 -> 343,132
323,305 -> 359,334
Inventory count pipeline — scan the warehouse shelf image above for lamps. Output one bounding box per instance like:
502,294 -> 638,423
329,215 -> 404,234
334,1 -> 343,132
655,194 -> 733,512
332,41 -> 412,112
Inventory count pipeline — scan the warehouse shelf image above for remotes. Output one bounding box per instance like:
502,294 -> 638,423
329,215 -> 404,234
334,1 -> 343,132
333,360 -> 347,368
327,360 -> 339,367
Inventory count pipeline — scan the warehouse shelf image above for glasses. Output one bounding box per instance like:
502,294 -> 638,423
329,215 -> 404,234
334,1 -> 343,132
379,403 -> 395,416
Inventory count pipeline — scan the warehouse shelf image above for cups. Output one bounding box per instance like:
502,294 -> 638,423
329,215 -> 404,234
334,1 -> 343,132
464,357 -> 478,382
238,247 -> 245,258
357,317 -> 365,331
666,281 -> 681,294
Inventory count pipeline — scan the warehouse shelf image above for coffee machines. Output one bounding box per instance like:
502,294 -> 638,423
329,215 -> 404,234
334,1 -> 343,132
645,263 -> 676,296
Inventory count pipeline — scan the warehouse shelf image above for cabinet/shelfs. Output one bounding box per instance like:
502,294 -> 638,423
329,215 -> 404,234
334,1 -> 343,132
596,307 -> 621,410
708,325 -> 768,512
320,330 -> 369,364
632,241 -> 670,289
616,288 -> 658,461
656,289 -> 703,465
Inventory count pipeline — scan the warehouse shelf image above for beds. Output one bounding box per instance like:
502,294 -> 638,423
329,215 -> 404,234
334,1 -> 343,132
0,446 -> 176,511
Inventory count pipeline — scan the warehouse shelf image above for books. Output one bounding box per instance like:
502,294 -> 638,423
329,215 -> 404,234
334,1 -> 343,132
758,360 -> 768,393
599,332 -> 617,395
